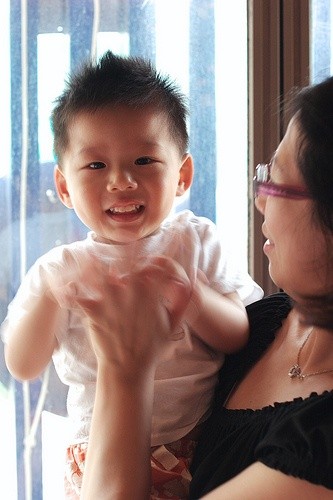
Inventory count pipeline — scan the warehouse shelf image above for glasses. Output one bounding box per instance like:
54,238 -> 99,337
253,160 -> 310,202
